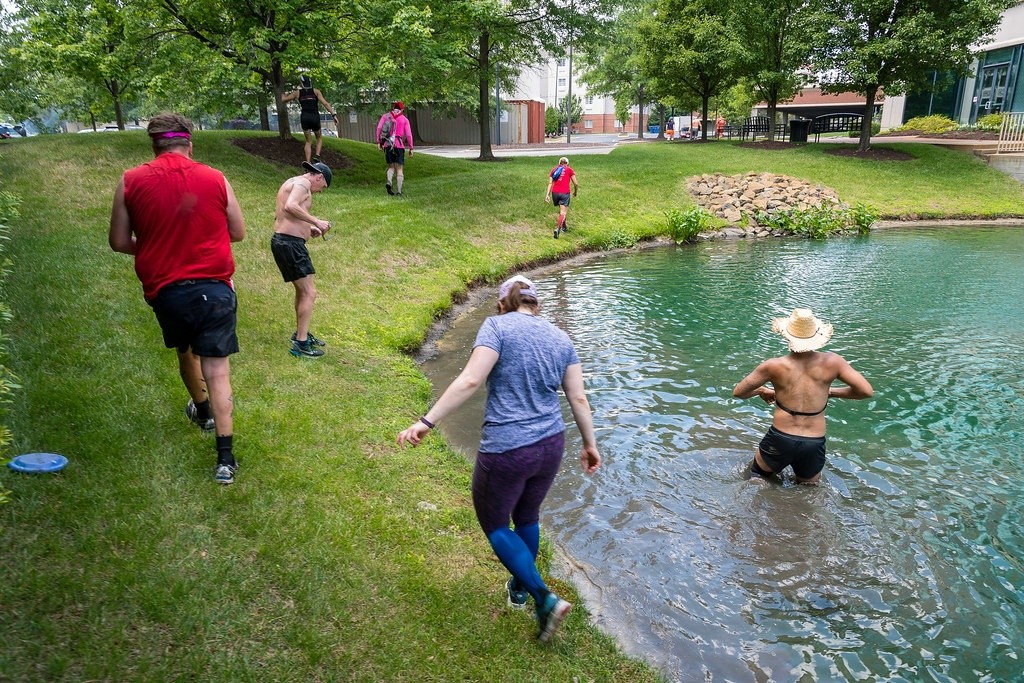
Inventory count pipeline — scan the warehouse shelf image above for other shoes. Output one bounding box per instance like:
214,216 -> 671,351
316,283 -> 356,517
554,228 -> 559,240
561,226 -> 567,231
386,181 -> 394,195
396,192 -> 402,196
313,155 -> 322,161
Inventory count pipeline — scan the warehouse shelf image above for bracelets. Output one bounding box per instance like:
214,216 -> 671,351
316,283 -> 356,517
332,114 -> 336,117
420,417 -> 435,428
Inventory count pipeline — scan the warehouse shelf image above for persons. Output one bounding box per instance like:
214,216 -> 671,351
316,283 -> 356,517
546,157 -> 578,239
692,116 -> 702,139
395,276 -> 601,644
715,115 -> 726,138
108,115 -> 244,486
666,119 -> 675,140
733,308 -> 873,485
270,161 -> 332,359
281,76 -> 339,165
376,102 -> 413,195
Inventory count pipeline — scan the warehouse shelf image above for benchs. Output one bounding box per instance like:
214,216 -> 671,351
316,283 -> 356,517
742,116 -> 786,142
813,113 -> 864,144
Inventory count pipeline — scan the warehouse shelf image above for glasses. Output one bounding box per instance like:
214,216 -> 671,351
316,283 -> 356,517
321,230 -> 336,241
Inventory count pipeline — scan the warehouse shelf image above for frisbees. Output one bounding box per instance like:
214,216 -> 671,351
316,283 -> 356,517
6,452 -> 69,473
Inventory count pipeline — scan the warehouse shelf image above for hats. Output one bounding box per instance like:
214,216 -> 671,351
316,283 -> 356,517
302,161 -> 332,187
394,102 -> 405,109
300,75 -> 312,89
498,275 -> 536,299
773,309 -> 833,353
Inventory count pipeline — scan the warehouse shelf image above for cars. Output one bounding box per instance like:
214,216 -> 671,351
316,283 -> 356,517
0,123 -> 35,140
77,125 -> 146,133
321,127 -> 337,138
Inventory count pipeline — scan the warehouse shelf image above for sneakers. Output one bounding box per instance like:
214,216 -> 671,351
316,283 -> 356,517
289,339 -> 325,358
290,332 -> 325,346
536,589 -> 571,643
215,460 -> 240,484
187,398 -> 215,430
505,577 -> 527,610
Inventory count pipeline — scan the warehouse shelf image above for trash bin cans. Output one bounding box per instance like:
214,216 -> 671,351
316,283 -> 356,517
649,126 -> 659,133
790,119 -> 812,142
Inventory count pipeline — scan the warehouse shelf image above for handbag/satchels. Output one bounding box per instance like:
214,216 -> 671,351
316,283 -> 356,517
380,113 -> 391,142
552,166 -> 564,179
